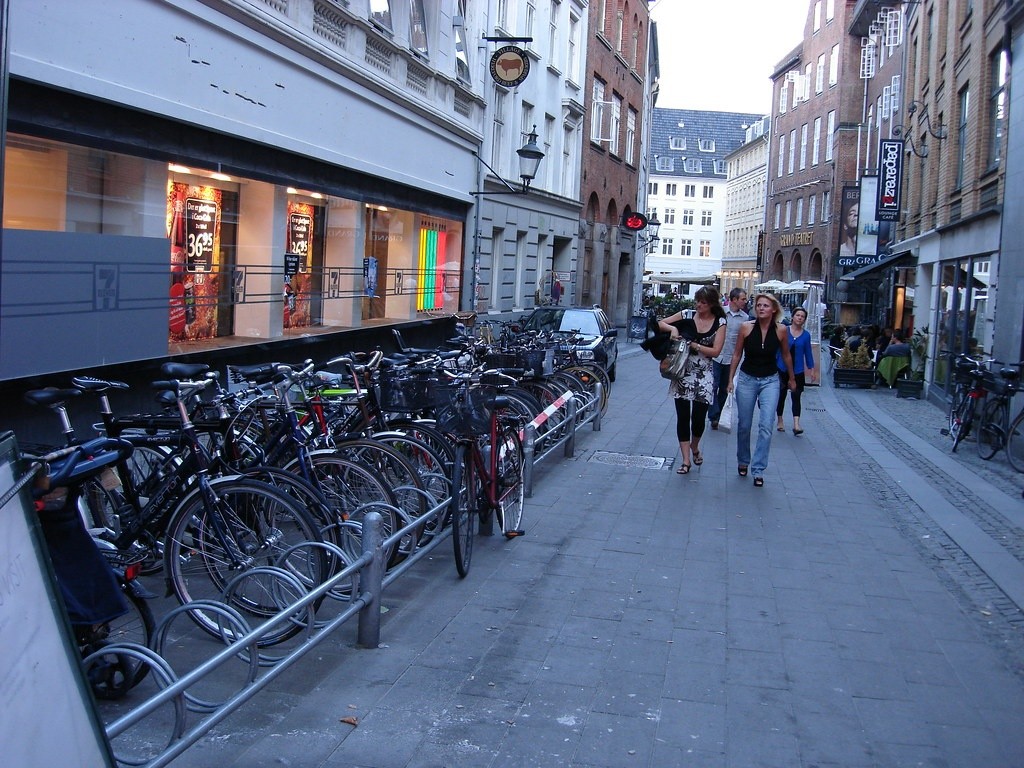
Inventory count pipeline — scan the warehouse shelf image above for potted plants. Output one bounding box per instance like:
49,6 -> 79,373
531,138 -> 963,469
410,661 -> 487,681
896,323 -> 931,399
833,338 -> 875,388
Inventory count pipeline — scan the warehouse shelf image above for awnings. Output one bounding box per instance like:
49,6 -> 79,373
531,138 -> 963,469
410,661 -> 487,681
839,250 -> 911,281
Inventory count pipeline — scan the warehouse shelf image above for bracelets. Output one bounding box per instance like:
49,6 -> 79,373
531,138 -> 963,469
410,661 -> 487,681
696,344 -> 701,351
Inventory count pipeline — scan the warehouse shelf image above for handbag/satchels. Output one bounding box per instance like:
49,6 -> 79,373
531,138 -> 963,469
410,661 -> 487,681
659,309 -> 692,380
717,393 -> 733,434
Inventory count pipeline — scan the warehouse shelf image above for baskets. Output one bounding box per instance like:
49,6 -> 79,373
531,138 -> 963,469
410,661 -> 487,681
379,341 -> 564,438
453,311 -> 478,327
956,362 -> 1021,397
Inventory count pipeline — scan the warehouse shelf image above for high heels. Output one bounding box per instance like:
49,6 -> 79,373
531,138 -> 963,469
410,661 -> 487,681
676,462 -> 692,474
693,451 -> 703,466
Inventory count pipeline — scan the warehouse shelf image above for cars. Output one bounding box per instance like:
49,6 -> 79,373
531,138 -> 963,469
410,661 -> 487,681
521,304 -> 618,382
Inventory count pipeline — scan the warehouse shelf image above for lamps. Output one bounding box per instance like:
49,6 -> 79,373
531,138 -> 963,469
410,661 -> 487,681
471,124 -> 545,196
637,210 -> 662,249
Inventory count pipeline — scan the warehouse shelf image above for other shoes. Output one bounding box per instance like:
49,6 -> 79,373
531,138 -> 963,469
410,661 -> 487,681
711,420 -> 719,429
793,429 -> 804,436
777,428 -> 785,432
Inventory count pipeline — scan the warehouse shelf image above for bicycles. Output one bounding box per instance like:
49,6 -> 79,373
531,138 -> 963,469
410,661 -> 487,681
940,351 -> 1024,473
0,320 -> 611,700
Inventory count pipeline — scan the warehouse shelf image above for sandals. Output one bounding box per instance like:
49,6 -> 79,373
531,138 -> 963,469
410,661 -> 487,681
738,464 -> 748,476
754,476 -> 764,487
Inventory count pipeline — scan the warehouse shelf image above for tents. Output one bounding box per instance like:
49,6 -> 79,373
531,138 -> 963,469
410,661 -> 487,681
753,279 -> 824,295
642,266 -> 716,284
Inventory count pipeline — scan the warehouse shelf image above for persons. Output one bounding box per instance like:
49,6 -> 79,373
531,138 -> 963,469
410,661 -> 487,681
658,285 -> 728,474
840,200 -> 858,256
776,307 -> 816,437
882,329 -> 911,357
708,287 -> 750,430
728,294 -> 796,487
719,293 -> 729,306
643,296 -> 655,306
829,325 -> 875,359
